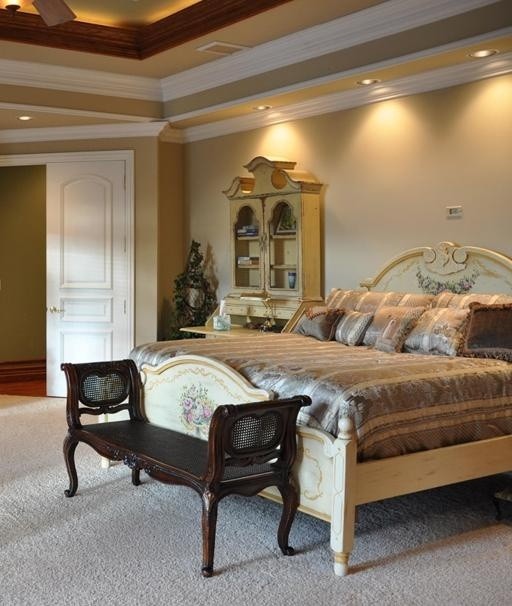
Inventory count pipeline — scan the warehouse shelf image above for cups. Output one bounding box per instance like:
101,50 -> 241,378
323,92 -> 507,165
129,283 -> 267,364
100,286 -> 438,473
288,272 -> 296,289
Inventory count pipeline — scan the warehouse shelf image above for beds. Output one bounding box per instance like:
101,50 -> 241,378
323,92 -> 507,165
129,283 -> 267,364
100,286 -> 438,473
130,241 -> 511,577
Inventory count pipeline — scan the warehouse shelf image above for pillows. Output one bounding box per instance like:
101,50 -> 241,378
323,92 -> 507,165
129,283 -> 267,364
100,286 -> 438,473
362,306 -> 426,353
404,307 -> 470,356
291,306 -> 345,342
325,288 -> 435,310
335,311 -> 374,347
432,291 -> 512,307
464,301 -> 512,362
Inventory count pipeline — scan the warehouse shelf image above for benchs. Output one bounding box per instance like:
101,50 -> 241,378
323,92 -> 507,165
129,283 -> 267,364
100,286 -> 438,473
61,359 -> 312,577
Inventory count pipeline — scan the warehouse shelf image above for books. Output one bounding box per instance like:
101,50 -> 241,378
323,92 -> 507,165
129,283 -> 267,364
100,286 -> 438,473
237,225 -> 258,236
238,260 -> 258,265
238,256 -> 259,260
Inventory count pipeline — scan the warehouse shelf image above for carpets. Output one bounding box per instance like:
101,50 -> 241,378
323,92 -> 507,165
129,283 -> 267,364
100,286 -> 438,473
1,392 -> 511,604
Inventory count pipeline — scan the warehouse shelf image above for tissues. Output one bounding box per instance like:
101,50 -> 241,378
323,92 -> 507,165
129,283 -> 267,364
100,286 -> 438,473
213,299 -> 232,331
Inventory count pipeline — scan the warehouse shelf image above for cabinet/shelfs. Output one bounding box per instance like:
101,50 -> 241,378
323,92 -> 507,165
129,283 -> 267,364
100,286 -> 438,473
178,156 -> 324,336
